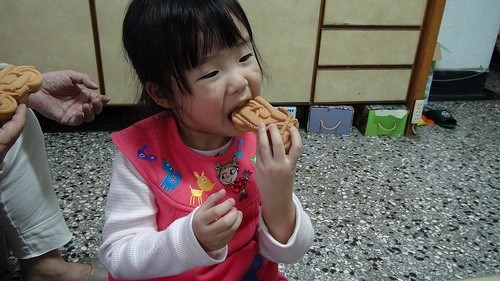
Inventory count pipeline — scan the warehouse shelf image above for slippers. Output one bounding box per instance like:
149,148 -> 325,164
424,109 -> 459,129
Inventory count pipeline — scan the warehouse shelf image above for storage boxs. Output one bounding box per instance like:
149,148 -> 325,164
363,105 -> 407,135
307,105 -> 353,134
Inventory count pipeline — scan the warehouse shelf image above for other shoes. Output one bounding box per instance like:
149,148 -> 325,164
87,263 -> 109,281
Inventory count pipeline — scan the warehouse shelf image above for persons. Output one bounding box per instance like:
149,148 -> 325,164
99,1 -> 314,280
0,63 -> 110,281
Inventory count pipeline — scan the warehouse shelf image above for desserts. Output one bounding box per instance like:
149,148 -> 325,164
231,95 -> 300,154
0,64 -> 43,121
